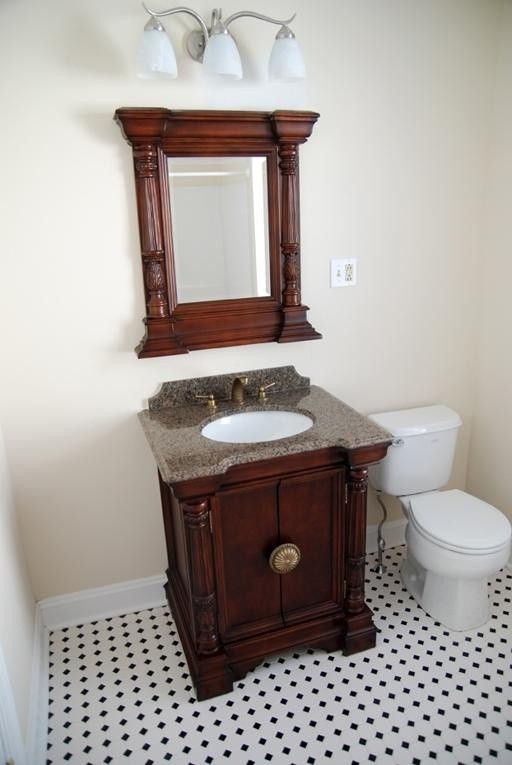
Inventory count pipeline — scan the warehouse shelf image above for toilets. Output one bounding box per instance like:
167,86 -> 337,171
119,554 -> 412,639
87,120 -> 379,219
368,405 -> 512,631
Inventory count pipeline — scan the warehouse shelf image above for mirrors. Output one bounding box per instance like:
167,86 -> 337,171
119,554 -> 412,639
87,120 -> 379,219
112,107 -> 322,360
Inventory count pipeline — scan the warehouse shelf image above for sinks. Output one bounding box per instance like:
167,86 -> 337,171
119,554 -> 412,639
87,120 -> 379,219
200,406 -> 316,442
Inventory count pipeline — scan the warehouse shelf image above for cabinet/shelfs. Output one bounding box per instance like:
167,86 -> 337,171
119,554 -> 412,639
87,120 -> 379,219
157,443 -> 391,702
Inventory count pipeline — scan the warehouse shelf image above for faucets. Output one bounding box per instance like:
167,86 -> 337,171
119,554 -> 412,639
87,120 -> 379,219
232,375 -> 248,403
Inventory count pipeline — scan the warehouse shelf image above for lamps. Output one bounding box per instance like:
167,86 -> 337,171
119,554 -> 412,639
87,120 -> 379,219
137,0 -> 305,83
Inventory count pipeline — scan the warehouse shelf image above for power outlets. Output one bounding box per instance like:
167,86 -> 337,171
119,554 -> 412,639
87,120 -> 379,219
331,257 -> 357,288
